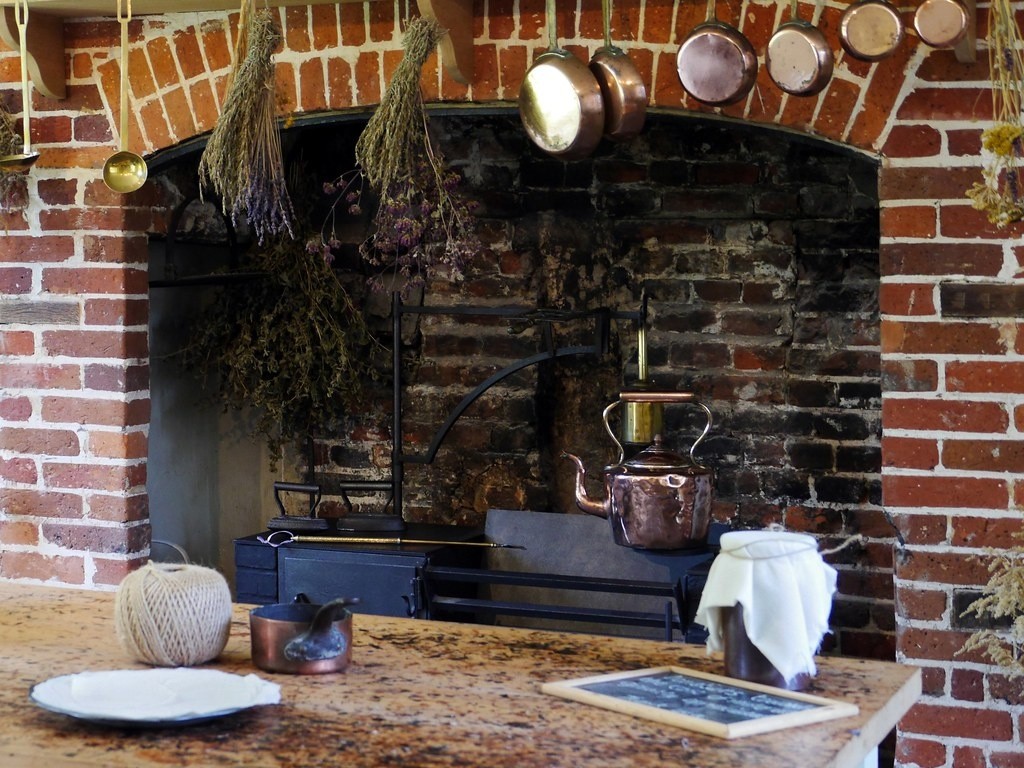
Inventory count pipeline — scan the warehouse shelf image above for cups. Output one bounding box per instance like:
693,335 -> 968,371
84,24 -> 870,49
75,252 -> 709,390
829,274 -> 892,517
718,603 -> 811,691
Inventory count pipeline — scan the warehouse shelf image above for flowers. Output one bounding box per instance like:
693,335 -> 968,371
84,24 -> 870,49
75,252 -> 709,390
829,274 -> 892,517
198,4 -> 298,247
0,93 -> 32,215
306,11 -> 494,301
964,0 -> 1024,229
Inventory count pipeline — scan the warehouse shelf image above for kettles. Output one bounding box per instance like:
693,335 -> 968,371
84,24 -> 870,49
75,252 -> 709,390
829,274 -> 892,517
561,392 -> 715,551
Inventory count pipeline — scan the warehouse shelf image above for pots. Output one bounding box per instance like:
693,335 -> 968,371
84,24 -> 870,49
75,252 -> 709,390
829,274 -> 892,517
249,591 -> 365,674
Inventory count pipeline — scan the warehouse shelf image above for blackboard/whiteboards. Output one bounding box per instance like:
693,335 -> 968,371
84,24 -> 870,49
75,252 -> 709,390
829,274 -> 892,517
538,664 -> 860,739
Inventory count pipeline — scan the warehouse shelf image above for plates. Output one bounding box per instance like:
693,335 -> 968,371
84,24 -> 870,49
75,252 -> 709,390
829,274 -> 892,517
30,664 -> 283,725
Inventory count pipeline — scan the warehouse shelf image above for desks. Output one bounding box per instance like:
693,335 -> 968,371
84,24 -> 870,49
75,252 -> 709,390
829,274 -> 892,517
0,585 -> 922,768
